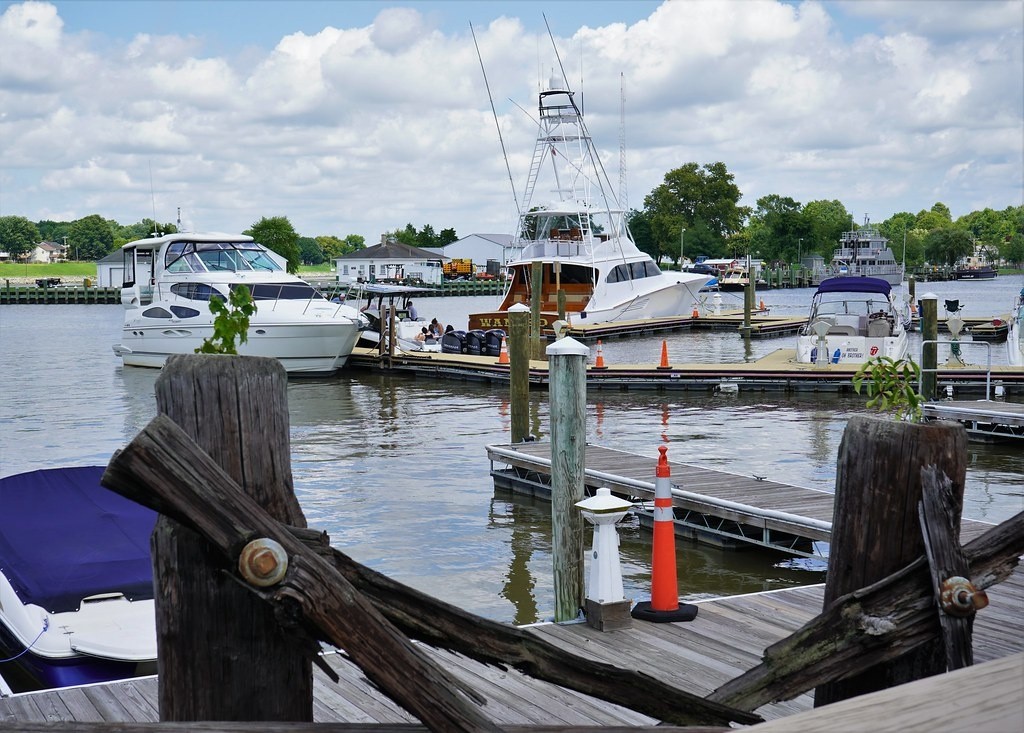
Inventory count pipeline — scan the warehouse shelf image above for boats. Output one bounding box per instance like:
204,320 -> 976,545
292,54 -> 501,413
111,159 -> 371,380
330,282 -> 444,350
468,12 -> 715,338
952,245 -> 999,280
795,208 -> 908,363
831,209 -> 902,285
719,264 -> 756,292
0,466 -> 162,691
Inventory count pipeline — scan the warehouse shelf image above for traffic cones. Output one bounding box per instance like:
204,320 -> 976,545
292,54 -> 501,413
591,340 -> 608,370
495,336 -> 510,364
692,303 -> 699,319
656,341 -> 672,370
759,297 -> 766,310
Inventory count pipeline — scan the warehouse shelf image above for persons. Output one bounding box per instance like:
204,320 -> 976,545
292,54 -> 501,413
322,293 -> 331,301
415,318 -> 454,341
332,293 -> 347,305
408,301 -> 418,321
